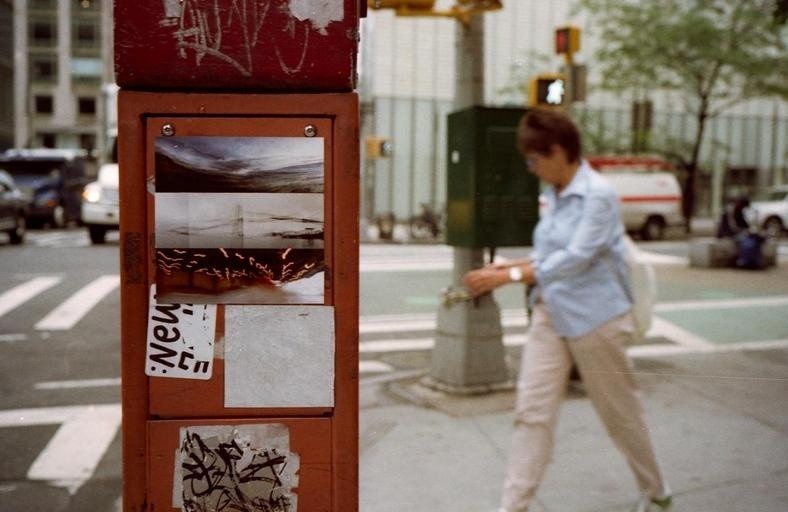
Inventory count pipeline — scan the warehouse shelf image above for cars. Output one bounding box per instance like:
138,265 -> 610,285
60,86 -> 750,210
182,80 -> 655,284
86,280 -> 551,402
1,146 -> 119,242
746,184 -> 788,241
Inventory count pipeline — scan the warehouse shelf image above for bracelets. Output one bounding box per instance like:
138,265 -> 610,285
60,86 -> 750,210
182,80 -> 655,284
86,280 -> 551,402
510,263 -> 525,283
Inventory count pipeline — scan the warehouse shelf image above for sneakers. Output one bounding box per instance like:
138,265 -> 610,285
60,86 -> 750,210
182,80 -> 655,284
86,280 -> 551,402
631,490 -> 673,512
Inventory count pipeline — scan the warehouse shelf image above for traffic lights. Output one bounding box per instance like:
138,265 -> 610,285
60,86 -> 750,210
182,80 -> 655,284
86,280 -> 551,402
529,75 -> 568,111
365,135 -> 394,161
555,26 -> 579,55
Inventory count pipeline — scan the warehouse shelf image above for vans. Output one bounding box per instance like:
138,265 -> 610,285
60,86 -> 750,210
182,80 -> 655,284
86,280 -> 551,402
582,155 -> 686,238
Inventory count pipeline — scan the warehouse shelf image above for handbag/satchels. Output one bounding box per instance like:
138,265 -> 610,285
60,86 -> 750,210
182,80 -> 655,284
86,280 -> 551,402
606,247 -> 658,346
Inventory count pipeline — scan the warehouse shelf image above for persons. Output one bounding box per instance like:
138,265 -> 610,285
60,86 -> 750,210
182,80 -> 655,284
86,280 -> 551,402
460,103 -> 675,512
717,189 -> 768,271
